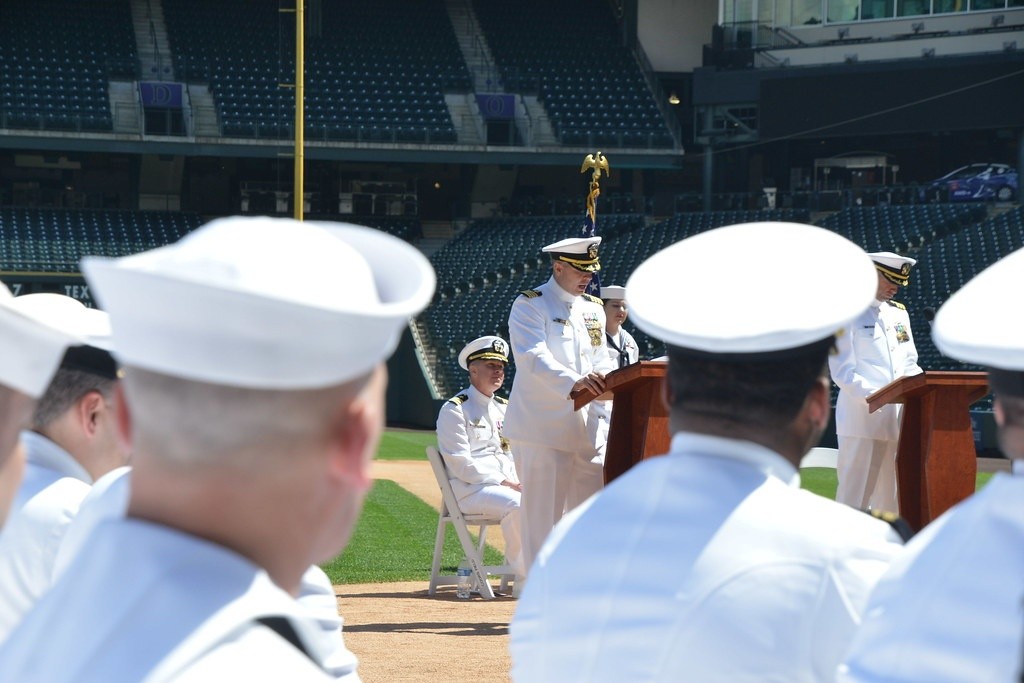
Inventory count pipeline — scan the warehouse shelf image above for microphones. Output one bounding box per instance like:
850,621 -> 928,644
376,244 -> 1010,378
923,306 -> 935,327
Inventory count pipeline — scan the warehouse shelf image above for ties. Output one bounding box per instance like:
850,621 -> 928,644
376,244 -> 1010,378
605,332 -> 630,369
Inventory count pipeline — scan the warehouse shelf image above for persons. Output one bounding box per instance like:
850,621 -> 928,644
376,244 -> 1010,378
438,336 -> 526,598
0,216 -> 438,683
507,236 -> 614,574
829,252 -> 924,514
508,221 -> 913,683
597,285 -> 640,370
835,244 -> 1024,683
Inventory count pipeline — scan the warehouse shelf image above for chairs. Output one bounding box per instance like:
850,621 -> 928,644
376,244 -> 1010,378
0,1 -> 1023,416
427,443 -> 516,601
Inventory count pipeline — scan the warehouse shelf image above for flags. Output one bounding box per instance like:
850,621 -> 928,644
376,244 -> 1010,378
578,190 -> 602,298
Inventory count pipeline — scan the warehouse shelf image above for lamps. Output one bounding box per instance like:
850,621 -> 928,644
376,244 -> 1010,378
845,51 -> 861,63
910,22 -> 923,33
835,27 -> 849,40
989,15 -> 1003,27
1002,40 -> 1018,51
921,48 -> 934,56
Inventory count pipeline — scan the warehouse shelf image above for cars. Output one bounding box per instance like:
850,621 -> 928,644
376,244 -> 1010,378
918,161 -> 1019,205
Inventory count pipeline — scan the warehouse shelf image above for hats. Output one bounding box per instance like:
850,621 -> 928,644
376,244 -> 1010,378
930,248 -> 1024,396
457,335 -> 511,371
618,218 -> 881,385
542,237 -> 602,273
79,213 -> 438,391
598,284 -> 627,301
865,249 -> 917,288
58,309 -> 117,381
0,281 -> 88,398
